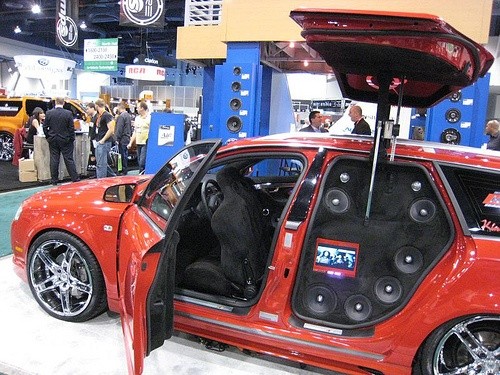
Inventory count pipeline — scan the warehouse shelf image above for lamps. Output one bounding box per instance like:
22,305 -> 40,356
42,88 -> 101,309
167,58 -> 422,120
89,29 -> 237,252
14,26 -> 22,33
79,7 -> 87,29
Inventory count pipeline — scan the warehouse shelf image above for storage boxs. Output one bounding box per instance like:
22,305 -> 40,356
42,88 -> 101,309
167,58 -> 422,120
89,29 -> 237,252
18,170 -> 38,182
19,157 -> 35,171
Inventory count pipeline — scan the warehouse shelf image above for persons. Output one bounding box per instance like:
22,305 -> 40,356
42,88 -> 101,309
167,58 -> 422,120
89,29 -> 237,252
44,97 -> 82,184
484,119 -> 500,151
87,101 -> 152,176
348,106 -> 371,135
20,107 -> 45,158
414,126 -> 425,141
91,99 -> 117,179
299,111 -> 329,133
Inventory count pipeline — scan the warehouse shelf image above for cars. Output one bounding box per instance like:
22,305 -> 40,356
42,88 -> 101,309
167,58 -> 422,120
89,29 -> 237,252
9,7 -> 500,375
0,95 -> 93,163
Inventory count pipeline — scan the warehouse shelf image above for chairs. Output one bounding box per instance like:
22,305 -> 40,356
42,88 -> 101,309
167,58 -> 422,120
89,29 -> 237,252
183,164 -> 275,301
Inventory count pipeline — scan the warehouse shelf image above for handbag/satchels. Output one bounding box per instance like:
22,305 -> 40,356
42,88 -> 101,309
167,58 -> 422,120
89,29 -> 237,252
114,149 -> 122,171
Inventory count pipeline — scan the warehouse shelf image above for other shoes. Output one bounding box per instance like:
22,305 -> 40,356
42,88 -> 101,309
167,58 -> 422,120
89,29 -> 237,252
89,175 -> 96,179
52,182 -> 57,185
117,172 -> 126,176
